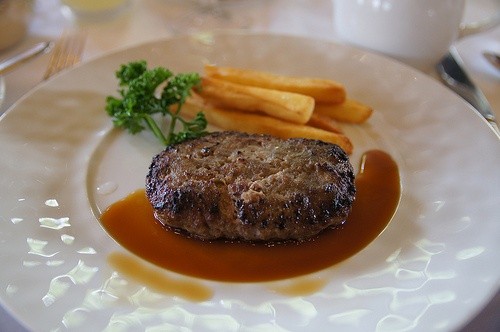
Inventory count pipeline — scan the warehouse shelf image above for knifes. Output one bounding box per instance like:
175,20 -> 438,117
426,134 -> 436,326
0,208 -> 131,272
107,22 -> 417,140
436,51 -> 493,121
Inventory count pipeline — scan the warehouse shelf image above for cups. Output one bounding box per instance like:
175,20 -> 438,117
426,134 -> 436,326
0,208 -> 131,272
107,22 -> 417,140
0,0 -> 34,50
335,0 -> 465,71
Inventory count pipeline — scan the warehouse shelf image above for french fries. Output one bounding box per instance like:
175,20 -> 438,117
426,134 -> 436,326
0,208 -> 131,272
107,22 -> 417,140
170,64 -> 374,156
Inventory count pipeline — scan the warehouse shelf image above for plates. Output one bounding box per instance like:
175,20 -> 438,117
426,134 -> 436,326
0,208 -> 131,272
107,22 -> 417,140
0,30 -> 499,331
449,27 -> 500,127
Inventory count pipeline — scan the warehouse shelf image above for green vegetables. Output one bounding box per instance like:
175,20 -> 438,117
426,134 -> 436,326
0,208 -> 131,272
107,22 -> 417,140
103,60 -> 208,146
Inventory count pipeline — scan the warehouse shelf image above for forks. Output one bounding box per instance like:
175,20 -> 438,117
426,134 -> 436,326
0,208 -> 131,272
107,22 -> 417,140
41,29 -> 88,79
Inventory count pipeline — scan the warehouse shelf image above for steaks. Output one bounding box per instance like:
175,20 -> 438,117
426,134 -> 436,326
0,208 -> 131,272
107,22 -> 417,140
145,131 -> 356,245
